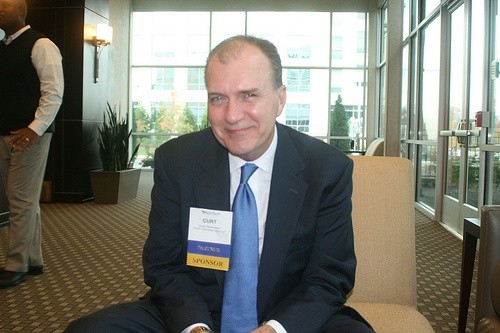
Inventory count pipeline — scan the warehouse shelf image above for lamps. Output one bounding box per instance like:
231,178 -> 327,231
84,23 -> 113,83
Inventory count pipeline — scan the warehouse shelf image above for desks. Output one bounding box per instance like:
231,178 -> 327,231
457,218 -> 481,333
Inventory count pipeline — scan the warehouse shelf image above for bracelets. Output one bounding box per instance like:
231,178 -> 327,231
191,327 -> 212,333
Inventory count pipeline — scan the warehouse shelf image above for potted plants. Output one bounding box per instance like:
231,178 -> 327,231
89,100 -> 141,204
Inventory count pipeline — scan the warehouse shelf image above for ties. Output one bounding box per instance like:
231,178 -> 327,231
220,164 -> 259,333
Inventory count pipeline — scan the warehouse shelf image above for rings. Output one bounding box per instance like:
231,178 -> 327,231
26,138 -> 29,142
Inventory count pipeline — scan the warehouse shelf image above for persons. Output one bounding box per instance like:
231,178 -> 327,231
62,35 -> 377,333
0,0 -> 64,289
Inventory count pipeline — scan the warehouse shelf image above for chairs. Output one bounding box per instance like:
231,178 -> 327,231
474,204 -> 500,333
331,156 -> 435,333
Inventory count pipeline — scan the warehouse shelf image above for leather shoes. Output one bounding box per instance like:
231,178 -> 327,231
0,271 -> 28,287
28,264 -> 43,274
